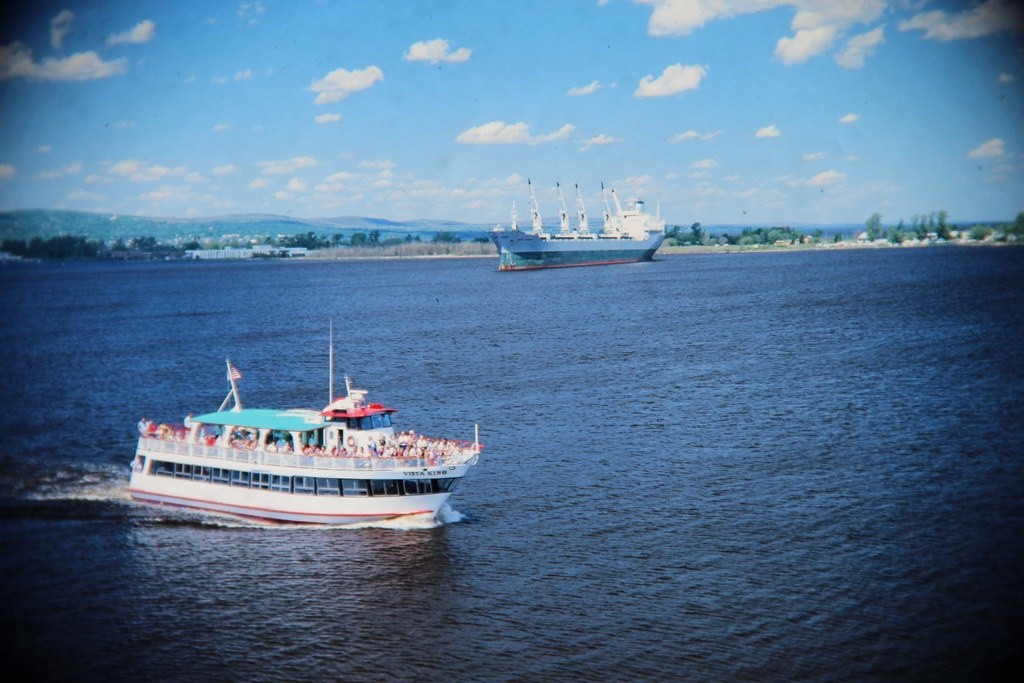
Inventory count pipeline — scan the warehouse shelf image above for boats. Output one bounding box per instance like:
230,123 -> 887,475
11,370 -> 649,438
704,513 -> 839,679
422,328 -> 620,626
126,315 -> 478,527
489,180 -> 668,272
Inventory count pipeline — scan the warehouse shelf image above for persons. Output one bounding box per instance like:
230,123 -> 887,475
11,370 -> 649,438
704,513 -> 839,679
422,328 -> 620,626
130,457 -> 143,473
267,431 -> 368,457
367,430 -> 473,467
137,413 -> 191,442
206,426 -> 260,451
